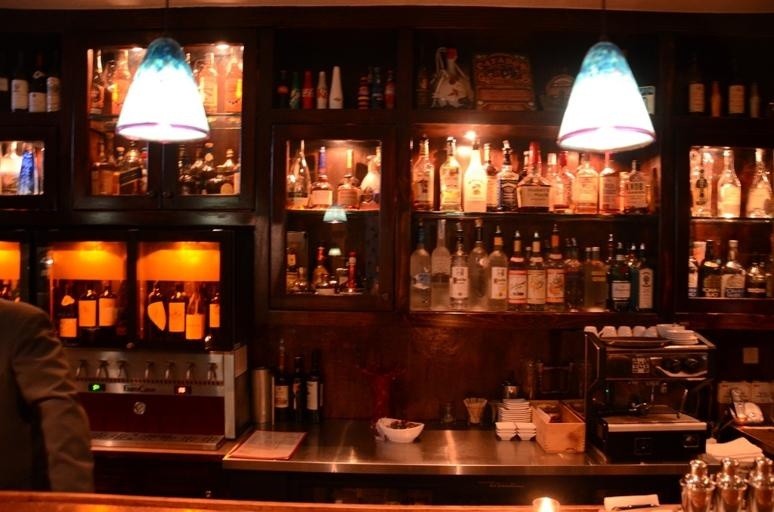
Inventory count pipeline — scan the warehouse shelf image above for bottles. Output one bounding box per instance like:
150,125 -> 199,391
28,51 -> 47,112
349,252 -> 356,289
482,142 -> 499,212
97,281 -> 120,347
746,260 -> 767,296
287,70 -> 301,109
698,239 -> 720,298
726,53 -> 746,119
555,151 -> 575,213
516,150 -> 530,181
275,338 -> 292,425
634,241 -> 655,312
508,229 -> 529,311
316,72 -> 327,109
285,140 -> 311,208
0,46 -> 10,109
575,152 -> 598,214
582,248 -> 593,306
277,69 -> 290,109
709,61 -> 723,118
464,145 -> 486,213
199,53 -> 218,112
311,247 -> 330,286
89,140 -> 111,195
625,243 -> 642,269
58,283 -> 78,342
497,146 -> 518,210
358,76 -> 370,110
206,287 -> 221,346
287,355 -> 306,424
47,71 -> 63,112
718,150 -> 741,218
609,241 -> 634,311
598,151 -> 620,214
410,136 -> 435,211
304,347 -> 324,426
287,266 -> 314,294
624,159 -> 647,211
687,241 -> 700,296
485,224 -> 508,311
371,67 -> 385,110
113,139 -> 238,196
338,171 -> 360,208
79,283 -> 99,345
336,267 -> 349,294
688,60 -> 706,118
144,284 -> 165,347
691,155 -> 711,218
591,246 -> 608,305
604,242 -> 617,267
253,366 -> 275,428
186,287 -> 208,352
448,222 -> 470,309
408,216 -> 432,310
329,66 -> 343,110
165,282 -> 189,351
89,50 -> 103,114
434,48 -> 473,110
302,70 -> 314,109
383,70 -> 396,110
544,224 -> 567,311
311,145 -> 332,208
527,232 -> 547,311
470,220 -> 490,310
563,237 -> 583,308
17,150 -> 34,195
439,138 -> 461,211
367,265 -> 381,292
750,84 -> 764,119
11,43 -> 28,111
432,220 -> 452,310
544,152 -> 565,213
747,148 -> 773,219
722,240 -> 747,298
518,141 -> 550,213
223,61 -> 242,113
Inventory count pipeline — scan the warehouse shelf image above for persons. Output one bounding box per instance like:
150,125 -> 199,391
0,299 -> 94,494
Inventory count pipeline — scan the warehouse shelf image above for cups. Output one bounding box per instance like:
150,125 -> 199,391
438,400 -> 458,427
246,364 -> 275,430
617,325 -> 633,337
645,326 -> 661,338
583,326 -> 599,338
633,324 -> 647,338
599,325 -> 617,338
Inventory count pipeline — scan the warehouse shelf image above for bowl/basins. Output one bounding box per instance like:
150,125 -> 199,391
656,322 -> 678,340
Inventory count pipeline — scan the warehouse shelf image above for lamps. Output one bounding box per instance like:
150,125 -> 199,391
557,0 -> 655,153
322,206 -> 347,224
115,0 -> 209,142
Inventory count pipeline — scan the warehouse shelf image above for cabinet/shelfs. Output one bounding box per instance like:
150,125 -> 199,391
0,6 -> 255,494
223,6 -> 774,507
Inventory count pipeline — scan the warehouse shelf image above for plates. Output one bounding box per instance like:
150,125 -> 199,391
494,422 -> 517,441
377,418 -> 425,444
665,328 -> 699,346
515,423 -> 537,442
497,406 -> 532,423
502,398 -> 531,411
